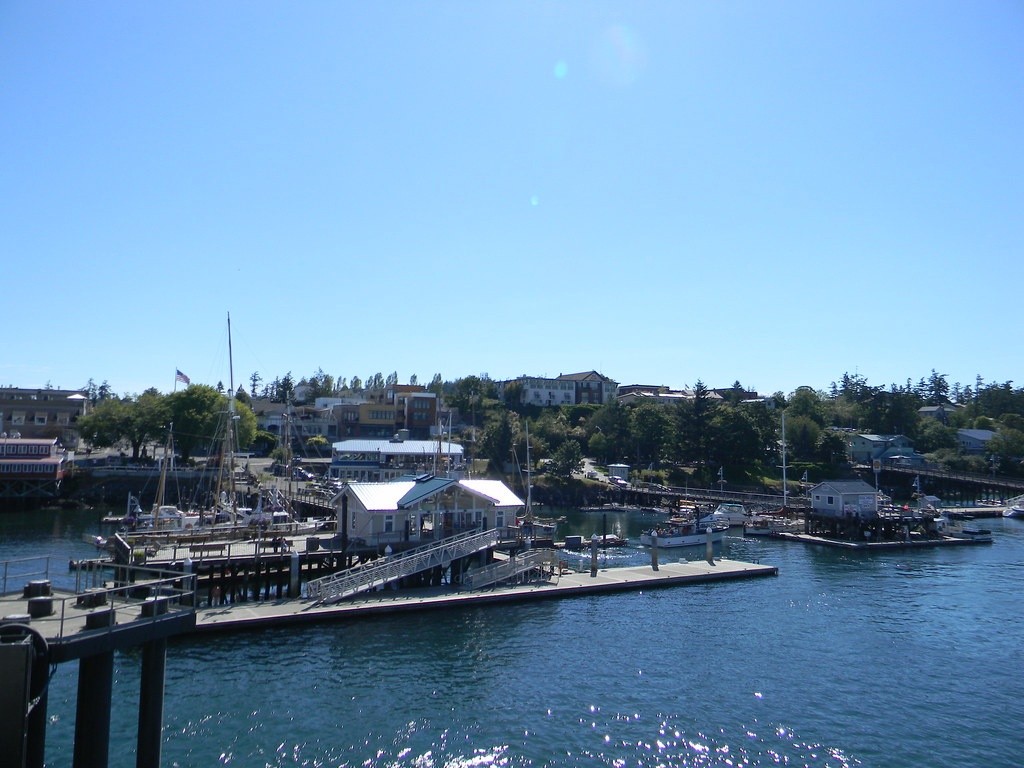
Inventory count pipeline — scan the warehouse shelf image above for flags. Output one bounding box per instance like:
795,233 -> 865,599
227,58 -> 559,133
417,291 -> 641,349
912,477 -> 918,486
801,471 -> 807,480
717,468 -> 722,475
648,463 -> 652,470
176,369 -> 190,384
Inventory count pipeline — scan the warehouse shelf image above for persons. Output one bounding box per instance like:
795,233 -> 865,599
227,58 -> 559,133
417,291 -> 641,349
471,521 -> 477,529
211,583 -> 221,609
272,535 -> 286,554
516,517 -> 520,527
647,524 -> 679,538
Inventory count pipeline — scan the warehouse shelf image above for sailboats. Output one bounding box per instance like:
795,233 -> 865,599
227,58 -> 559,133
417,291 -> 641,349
93,313 -> 1024,551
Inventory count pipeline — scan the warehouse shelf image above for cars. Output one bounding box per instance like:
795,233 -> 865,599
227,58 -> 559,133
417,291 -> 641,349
648,483 -> 671,493
608,475 -> 627,487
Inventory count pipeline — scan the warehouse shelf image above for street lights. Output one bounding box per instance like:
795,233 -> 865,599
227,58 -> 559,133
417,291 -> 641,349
801,469 -> 808,499
911,475 -> 920,517
775,408 -> 795,506
989,454 -> 995,482
717,466 -> 723,494
